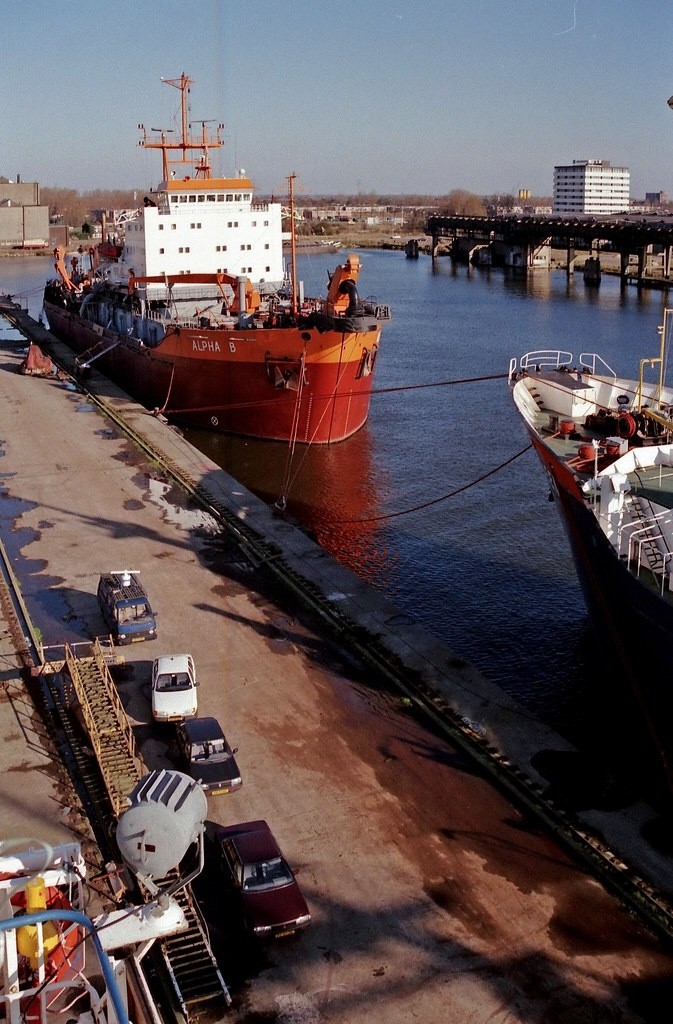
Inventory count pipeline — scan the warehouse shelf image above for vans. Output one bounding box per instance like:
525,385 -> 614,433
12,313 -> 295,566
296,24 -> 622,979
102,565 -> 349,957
96,568 -> 158,646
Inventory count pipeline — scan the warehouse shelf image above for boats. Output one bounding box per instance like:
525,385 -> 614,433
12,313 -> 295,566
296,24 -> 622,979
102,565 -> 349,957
40,70 -> 392,446
506,305 -> 673,639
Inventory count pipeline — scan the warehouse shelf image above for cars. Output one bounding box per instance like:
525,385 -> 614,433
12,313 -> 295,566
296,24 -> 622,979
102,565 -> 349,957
177,716 -> 244,798
214,818 -> 312,945
150,653 -> 200,723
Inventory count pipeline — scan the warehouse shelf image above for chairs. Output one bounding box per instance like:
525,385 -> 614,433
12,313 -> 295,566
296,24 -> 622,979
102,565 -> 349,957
177,674 -> 188,686
209,741 -> 224,755
238,866 -> 256,885
192,744 -> 205,756
157,675 -> 172,689
262,859 -> 282,877
